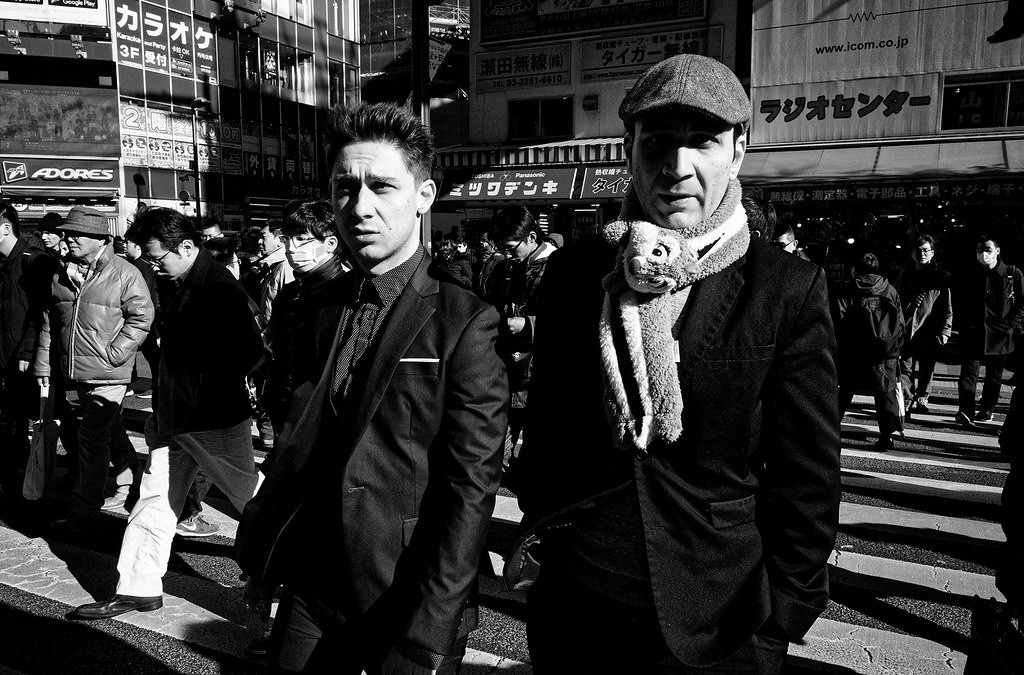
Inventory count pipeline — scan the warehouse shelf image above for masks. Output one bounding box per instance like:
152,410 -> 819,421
284,241 -> 332,273
977,252 -> 997,265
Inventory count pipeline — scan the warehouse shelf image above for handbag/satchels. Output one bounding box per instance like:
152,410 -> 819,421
23,384 -> 60,500
893,358 -> 905,428
1007,264 -> 1024,334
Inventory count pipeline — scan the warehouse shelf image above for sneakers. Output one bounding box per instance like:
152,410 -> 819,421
973,410 -> 993,422
955,410 -> 976,431
176,514 -> 220,536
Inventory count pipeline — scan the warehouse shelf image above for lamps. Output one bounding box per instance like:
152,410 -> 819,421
457,86 -> 470,104
244,9 -> 267,30
210,0 -> 235,19
7,29 -> 27,55
70,34 -> 87,58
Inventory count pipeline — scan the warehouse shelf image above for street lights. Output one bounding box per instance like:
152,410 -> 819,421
191,97 -> 212,232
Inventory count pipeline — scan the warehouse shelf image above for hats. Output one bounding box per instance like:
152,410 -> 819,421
37,213 -> 65,238
618,54 -> 752,123
56,207 -> 115,238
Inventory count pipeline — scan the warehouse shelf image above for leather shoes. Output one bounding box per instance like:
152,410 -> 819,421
75,594 -> 163,617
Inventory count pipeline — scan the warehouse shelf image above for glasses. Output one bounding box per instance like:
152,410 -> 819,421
279,235 -> 332,243
141,250 -> 171,266
201,233 -> 223,239
778,240 -> 796,249
494,237 -> 521,255
230,259 -> 242,265
915,249 -> 933,255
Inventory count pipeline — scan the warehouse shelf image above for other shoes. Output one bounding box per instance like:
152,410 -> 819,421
51,514 -> 100,531
245,628 -> 273,654
874,437 -> 894,447
917,397 -> 928,412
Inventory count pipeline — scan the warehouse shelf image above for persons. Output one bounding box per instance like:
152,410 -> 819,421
832,248 -> 906,451
893,233 -> 954,416
429,197 -> 565,476
0,201 -> 354,619
955,234 -> 1024,427
741,193 -> 811,262
995,373 -> 1024,634
498,53 -> 841,675
233,100 -> 510,675
